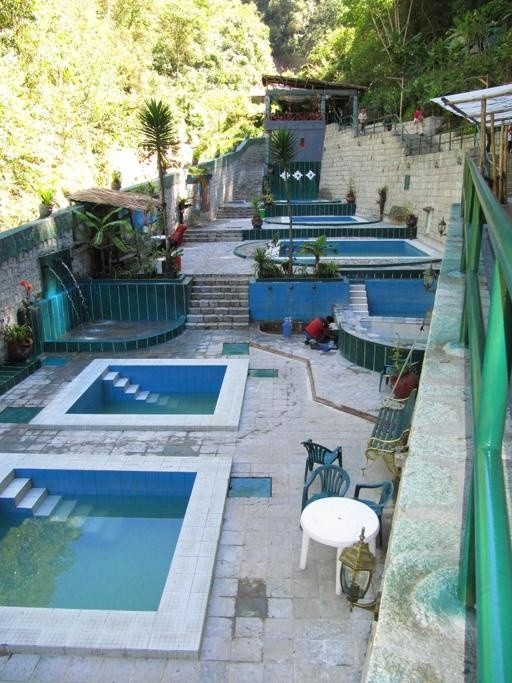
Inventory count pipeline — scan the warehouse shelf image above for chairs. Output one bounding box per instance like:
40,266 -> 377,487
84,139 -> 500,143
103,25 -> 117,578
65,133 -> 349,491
300,439 -> 342,484
350,480 -> 394,544
300,464 -> 350,531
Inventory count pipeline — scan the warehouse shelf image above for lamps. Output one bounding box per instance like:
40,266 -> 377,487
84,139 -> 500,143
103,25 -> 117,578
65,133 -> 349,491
339,527 -> 382,622
438,216 -> 446,238
423,262 -> 436,294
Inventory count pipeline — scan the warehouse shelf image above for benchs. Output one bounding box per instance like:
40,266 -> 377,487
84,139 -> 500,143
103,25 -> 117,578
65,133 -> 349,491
361,388 -> 418,477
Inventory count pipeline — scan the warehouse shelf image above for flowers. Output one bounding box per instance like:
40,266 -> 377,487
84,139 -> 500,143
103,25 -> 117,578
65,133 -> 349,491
19,279 -> 32,326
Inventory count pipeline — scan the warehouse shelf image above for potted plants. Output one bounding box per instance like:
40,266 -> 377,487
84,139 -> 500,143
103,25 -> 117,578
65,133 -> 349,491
38,189 -> 56,217
192,150 -> 199,166
3,323 -> 34,363
245,195 -> 268,228
110,170 -> 121,190
390,333 -> 419,398
405,207 -> 417,227
345,180 -> 355,204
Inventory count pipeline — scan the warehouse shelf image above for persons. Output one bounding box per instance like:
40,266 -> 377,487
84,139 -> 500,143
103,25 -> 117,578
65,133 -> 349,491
356,107 -> 368,131
303,314 -> 337,345
507,123 -> 511,153
412,105 -> 426,135
484,126 -> 497,153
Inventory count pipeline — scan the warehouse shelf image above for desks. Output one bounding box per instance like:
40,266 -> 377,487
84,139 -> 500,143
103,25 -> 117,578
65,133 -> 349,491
300,496 -> 380,596
